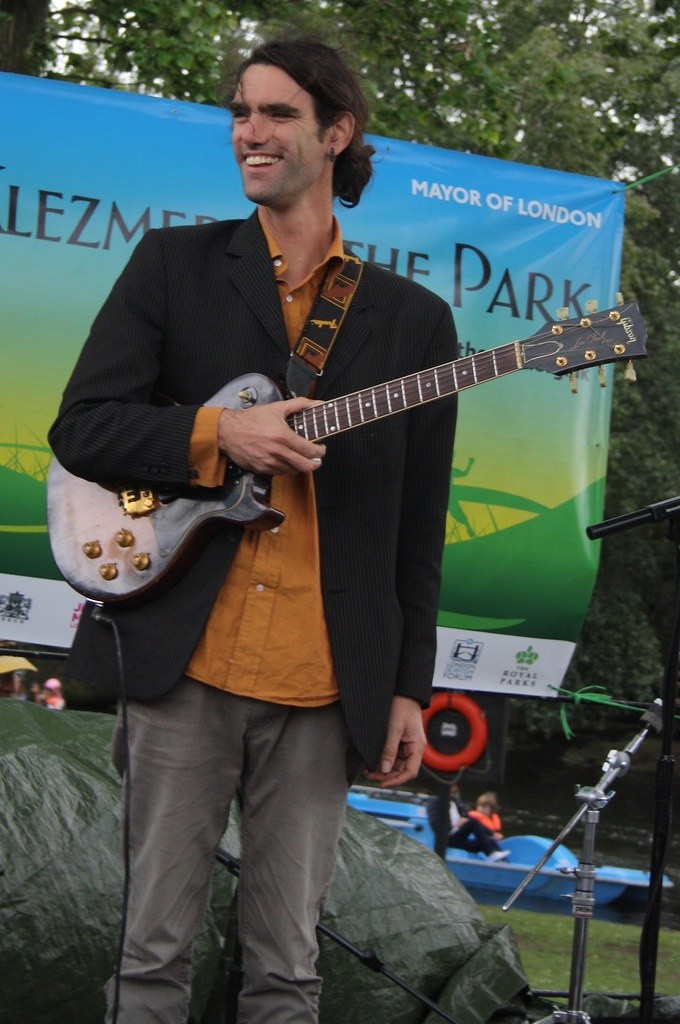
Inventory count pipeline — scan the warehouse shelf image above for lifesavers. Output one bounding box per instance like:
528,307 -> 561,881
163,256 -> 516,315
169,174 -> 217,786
421,693 -> 489,772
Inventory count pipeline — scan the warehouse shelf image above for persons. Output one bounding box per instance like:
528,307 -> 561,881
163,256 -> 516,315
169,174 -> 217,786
426,783 -> 511,862
48,41 -> 459,1023
10,675 -> 65,711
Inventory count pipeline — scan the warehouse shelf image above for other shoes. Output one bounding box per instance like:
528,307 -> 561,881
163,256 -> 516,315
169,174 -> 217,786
487,849 -> 511,862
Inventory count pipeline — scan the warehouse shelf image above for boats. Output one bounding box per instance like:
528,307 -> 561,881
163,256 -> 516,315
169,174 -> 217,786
347,785 -> 675,912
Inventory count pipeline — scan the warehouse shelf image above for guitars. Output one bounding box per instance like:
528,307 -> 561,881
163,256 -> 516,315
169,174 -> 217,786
47,291 -> 649,614
